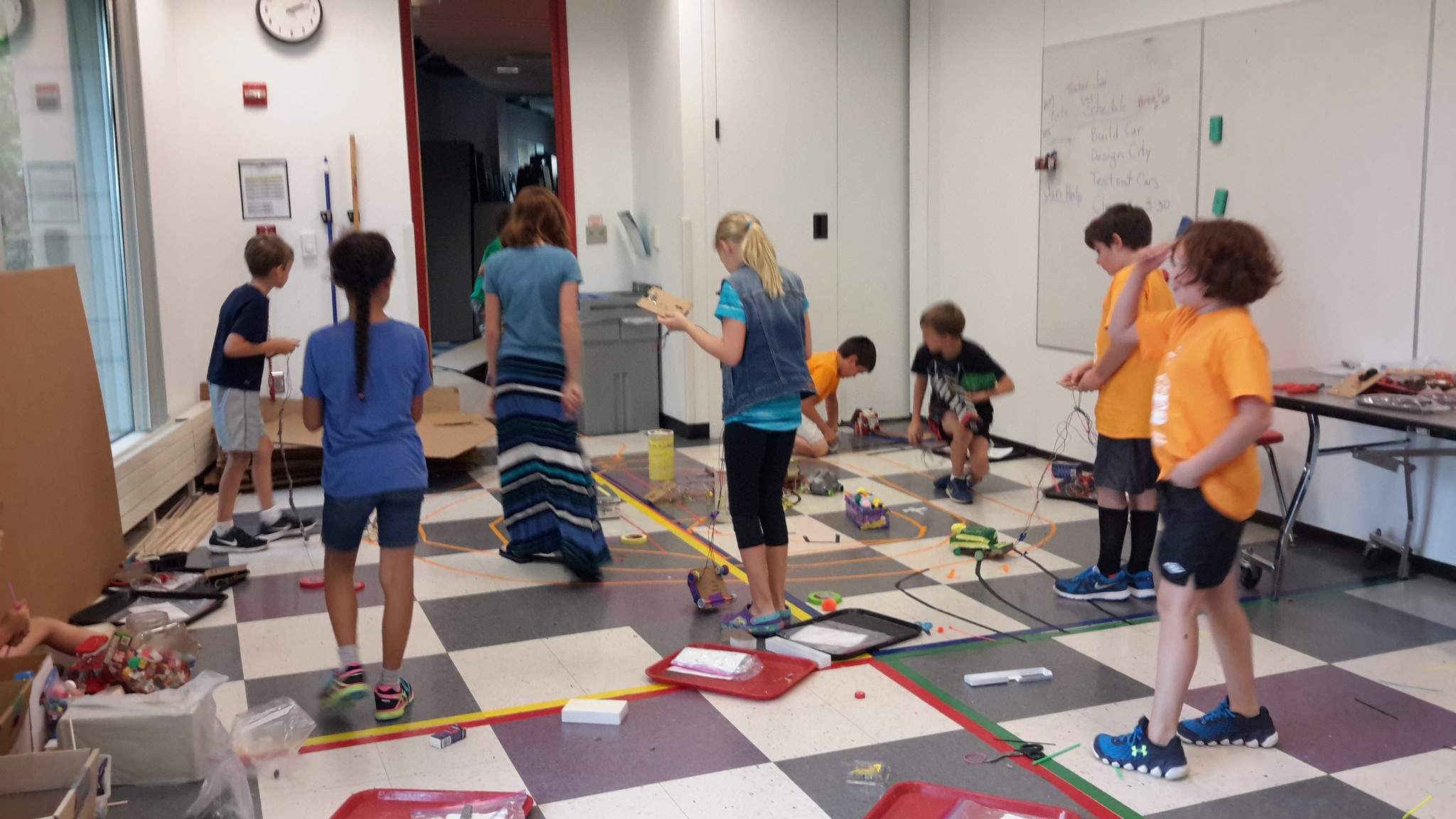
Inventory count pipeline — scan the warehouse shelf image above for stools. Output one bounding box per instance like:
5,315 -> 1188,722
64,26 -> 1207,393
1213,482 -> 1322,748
1252,427 -> 1297,542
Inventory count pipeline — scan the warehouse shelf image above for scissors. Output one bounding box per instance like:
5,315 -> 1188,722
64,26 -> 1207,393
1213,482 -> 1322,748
985,743 -> 1046,763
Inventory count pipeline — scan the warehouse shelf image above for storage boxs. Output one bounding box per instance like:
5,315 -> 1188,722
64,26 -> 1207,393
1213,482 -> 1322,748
0,746 -> 100,819
0,651 -> 57,756
102,609 -> 200,697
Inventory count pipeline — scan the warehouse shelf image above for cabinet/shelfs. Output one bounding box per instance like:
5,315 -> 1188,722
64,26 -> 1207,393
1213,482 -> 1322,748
576,290 -> 662,437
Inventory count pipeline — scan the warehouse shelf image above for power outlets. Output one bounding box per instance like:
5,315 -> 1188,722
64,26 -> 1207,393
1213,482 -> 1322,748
270,370 -> 286,393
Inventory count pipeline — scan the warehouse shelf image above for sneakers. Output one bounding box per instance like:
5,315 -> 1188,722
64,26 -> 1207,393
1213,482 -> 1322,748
1053,569 -> 1134,602
1178,697 -> 1278,749
257,513 -> 317,544
932,472 -> 954,491
207,528 -> 269,557
1122,563 -> 1159,600
1091,714 -> 1187,789
943,478 -> 974,503
370,678 -> 414,723
314,661 -> 369,718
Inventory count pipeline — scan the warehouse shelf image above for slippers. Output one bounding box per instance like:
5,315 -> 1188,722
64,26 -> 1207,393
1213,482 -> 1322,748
496,540 -> 531,563
573,559 -> 604,585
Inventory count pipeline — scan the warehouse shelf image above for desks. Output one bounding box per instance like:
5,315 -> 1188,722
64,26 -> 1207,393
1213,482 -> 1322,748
1237,364 -> 1456,601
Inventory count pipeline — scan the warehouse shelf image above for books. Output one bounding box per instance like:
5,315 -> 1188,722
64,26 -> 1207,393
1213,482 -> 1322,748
636,285 -> 693,320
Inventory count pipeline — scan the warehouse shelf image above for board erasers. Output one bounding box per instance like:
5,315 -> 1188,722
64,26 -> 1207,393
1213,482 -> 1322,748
1212,188 -> 1229,217
1176,215 -> 1192,240
1207,113 -> 1224,145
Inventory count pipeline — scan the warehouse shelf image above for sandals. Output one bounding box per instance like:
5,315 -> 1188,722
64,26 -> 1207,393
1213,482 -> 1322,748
718,604 -> 787,636
771,604 -> 791,631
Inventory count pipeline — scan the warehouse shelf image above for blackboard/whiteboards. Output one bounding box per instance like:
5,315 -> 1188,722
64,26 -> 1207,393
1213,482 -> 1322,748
1034,1 -> 1439,385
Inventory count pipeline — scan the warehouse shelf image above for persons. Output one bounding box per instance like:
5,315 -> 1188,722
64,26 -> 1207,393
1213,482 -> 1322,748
907,300 -> 1015,505
790,336 -> 876,459
1089,218 -> 1278,783
205,234 -> 320,551
656,209 -> 817,636
482,186 -> 613,586
471,207 -> 513,342
0,506 -> 114,659
1051,201 -> 1178,601
301,230 -> 435,722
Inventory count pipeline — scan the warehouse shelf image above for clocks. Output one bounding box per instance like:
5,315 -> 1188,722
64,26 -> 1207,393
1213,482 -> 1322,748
1,0 -> 35,43
255,0 -> 323,44
